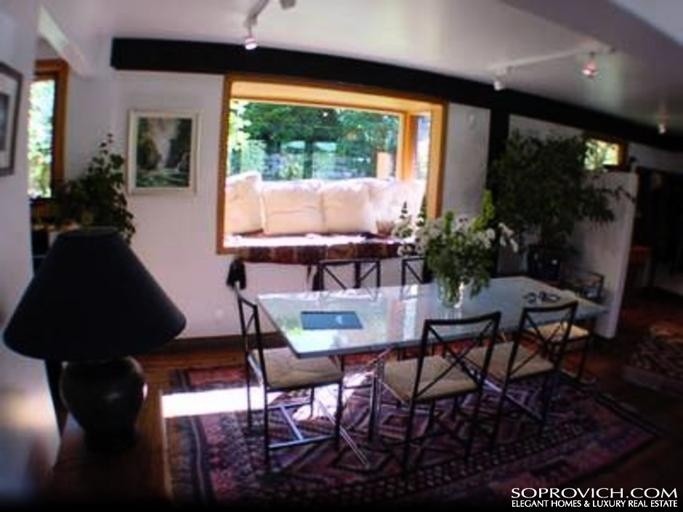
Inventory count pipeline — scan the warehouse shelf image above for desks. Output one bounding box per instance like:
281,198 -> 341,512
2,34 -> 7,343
49,371 -> 173,511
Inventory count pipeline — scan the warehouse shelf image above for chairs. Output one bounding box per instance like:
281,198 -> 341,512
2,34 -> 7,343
222,250 -> 610,484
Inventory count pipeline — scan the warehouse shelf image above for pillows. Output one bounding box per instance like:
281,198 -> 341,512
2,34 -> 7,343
224,168 -> 263,235
366,174 -> 427,231
260,179 -> 325,233
313,175 -> 379,237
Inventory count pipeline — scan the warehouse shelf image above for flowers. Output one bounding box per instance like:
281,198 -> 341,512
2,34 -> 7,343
392,186 -> 520,300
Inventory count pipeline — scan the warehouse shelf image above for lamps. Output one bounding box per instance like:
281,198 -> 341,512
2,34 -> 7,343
485,46 -> 615,94
242,0 -> 298,52
2,226 -> 188,452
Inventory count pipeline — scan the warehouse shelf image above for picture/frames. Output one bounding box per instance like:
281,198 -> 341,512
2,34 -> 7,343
1,61 -> 24,178
125,106 -> 205,199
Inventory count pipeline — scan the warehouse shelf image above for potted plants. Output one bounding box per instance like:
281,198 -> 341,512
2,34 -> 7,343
483,128 -> 642,282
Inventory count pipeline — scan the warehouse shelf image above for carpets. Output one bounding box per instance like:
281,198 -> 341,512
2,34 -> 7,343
174,338 -> 681,512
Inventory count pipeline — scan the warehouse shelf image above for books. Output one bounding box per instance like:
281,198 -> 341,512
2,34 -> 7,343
300,310 -> 361,330
